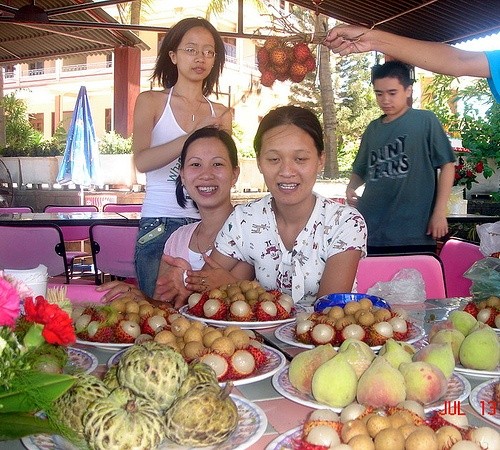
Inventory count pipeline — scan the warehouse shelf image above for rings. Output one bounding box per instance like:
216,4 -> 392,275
127,285 -> 132,293
201,277 -> 205,285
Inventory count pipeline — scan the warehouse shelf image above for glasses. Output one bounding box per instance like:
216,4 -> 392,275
176,47 -> 218,57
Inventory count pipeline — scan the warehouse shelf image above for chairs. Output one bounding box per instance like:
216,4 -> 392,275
0,203 -> 143,304
356,237 -> 484,301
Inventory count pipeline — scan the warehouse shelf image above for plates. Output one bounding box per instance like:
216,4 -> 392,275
492,327 -> 500,332
264,425 -> 307,450
469,379 -> 500,427
21,393 -> 268,450
107,344 -> 287,387
178,303 -> 309,329
76,337 -> 133,350
275,321 -> 427,351
271,364 -> 472,412
68,347 -> 98,377
452,363 -> 500,376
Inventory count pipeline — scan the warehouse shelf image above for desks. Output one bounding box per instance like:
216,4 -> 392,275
117,212 -> 140,226
0,304 -> 500,450
447,213 -> 500,240
0,212 -> 128,225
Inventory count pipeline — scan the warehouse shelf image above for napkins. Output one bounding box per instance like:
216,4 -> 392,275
3,262 -> 49,300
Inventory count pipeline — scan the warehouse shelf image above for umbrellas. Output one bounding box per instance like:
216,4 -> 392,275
56,85 -> 98,205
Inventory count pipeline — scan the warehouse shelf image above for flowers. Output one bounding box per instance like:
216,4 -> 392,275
453,159 -> 497,189
0,276 -> 88,445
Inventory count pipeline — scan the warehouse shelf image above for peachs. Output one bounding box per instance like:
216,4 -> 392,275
287,310 -> 500,410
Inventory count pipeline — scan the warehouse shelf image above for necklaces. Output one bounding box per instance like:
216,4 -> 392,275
197,230 -> 212,254
173,88 -> 203,121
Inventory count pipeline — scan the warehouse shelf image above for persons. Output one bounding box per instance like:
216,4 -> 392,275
346,60 -> 457,253
96,126 -> 240,307
133,17 -> 232,297
324,23 -> 500,105
155,106 -> 369,307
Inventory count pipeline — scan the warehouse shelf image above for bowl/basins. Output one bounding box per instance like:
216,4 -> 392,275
314,293 -> 391,312
